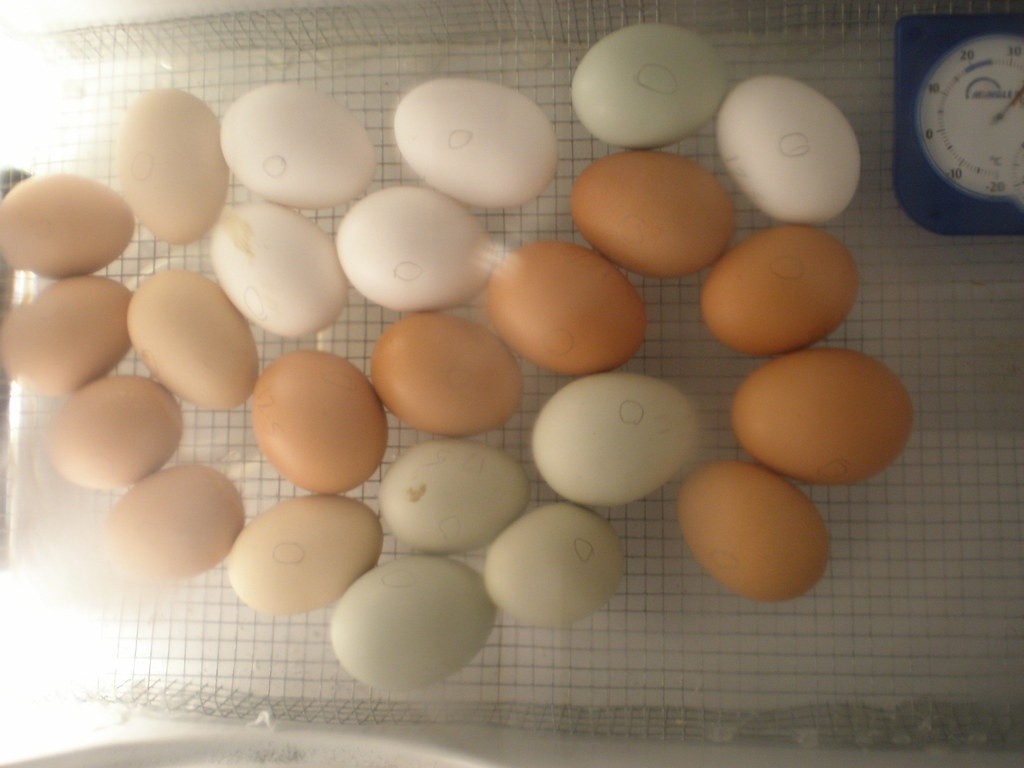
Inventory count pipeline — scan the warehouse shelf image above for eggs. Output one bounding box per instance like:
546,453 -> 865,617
2,25 -> 913,694
